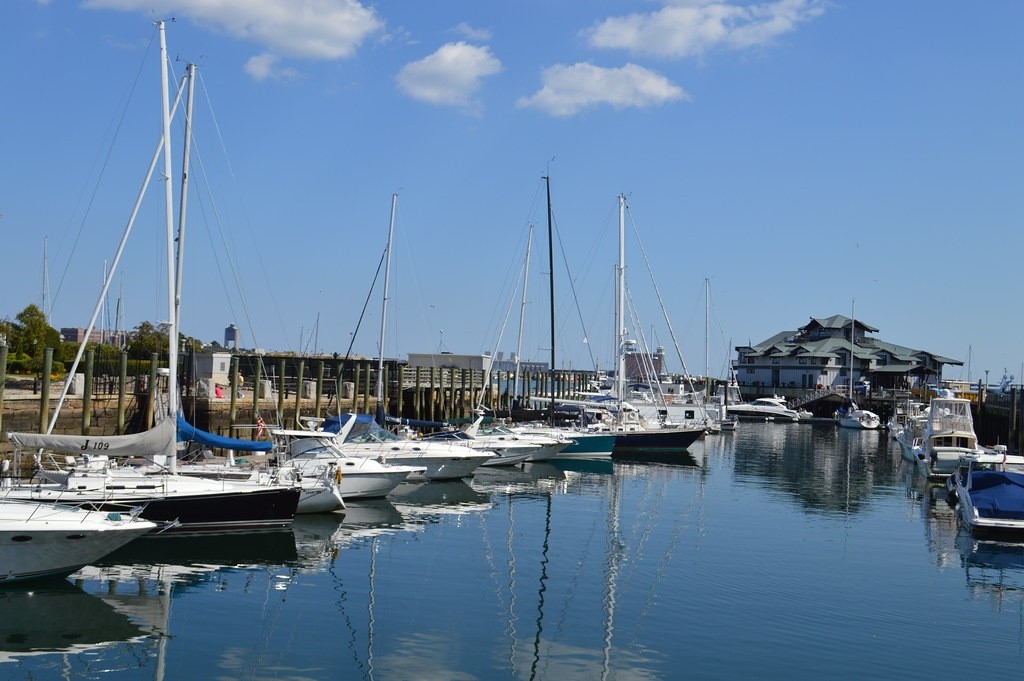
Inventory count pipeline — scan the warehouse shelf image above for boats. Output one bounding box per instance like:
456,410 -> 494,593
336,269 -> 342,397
0,579 -> 178,681
913,388 -> 984,480
716,394 -> 813,418
293,462 -> 569,573
948,444 -> 1024,547
887,392 -> 944,463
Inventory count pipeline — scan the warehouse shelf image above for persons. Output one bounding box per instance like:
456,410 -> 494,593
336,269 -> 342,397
237,372 -> 245,399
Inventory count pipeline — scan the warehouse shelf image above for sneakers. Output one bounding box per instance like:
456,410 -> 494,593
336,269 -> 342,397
242,395 -> 245,398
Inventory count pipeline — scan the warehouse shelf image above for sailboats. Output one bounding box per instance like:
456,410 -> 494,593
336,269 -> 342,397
70,526 -> 299,681
1,18 -> 738,585
838,300 -> 881,430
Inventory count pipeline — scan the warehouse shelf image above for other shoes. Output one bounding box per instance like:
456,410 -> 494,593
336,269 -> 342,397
930,465 -> 933,468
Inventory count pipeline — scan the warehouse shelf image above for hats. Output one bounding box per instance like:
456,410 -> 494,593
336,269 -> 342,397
238,373 -> 241,375
